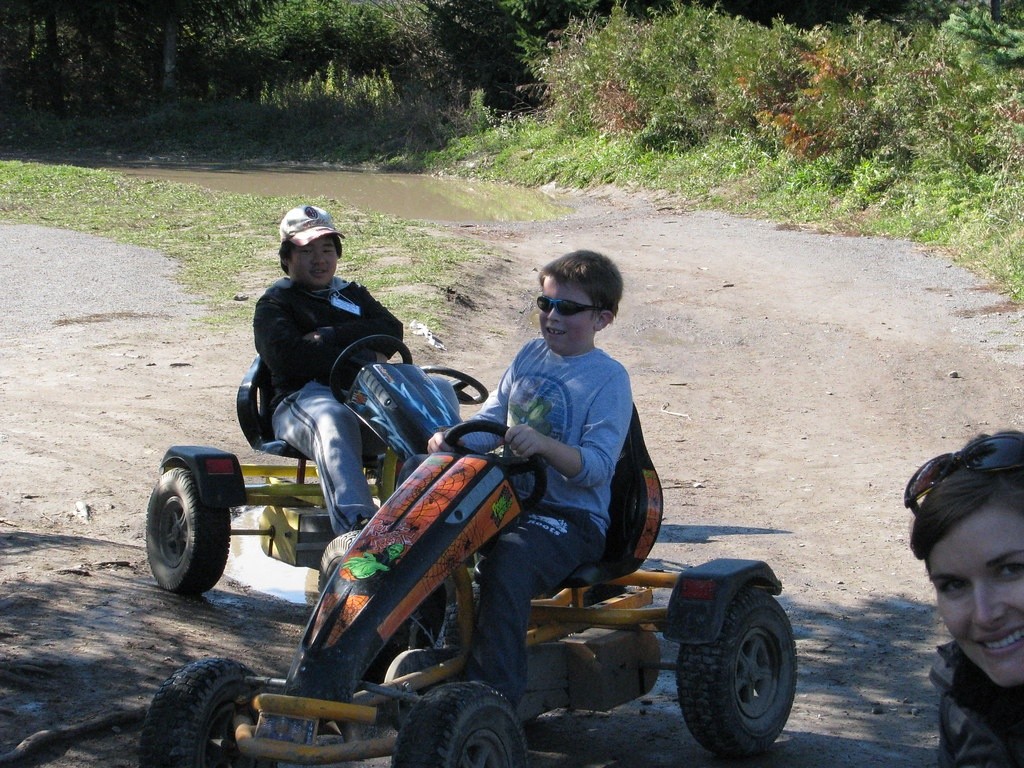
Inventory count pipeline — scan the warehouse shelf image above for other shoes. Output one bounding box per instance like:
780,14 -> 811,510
352,514 -> 372,532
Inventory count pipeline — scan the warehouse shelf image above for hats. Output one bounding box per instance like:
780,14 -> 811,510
279,206 -> 346,247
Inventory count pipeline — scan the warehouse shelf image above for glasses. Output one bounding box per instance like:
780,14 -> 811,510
537,296 -> 605,317
904,433 -> 1024,518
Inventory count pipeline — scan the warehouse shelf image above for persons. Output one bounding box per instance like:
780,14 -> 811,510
252,203 -> 463,536
395,248 -> 634,705
903,425 -> 1024,768
